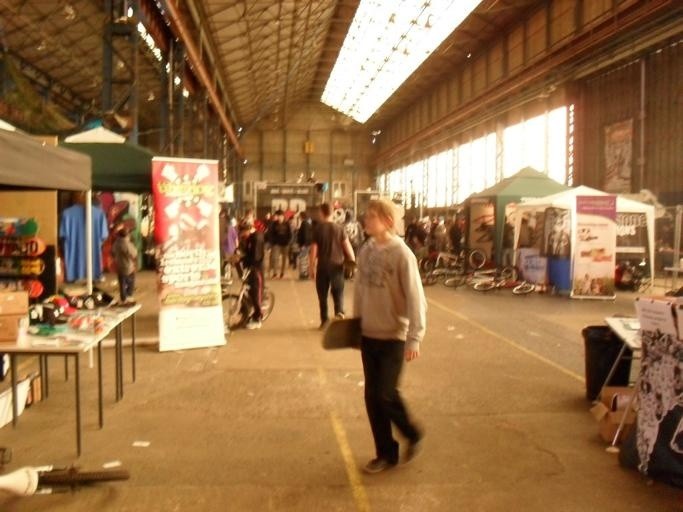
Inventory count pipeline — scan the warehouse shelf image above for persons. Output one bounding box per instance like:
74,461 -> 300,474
108,227 -> 138,306
501,216 -> 528,270
221,200 -> 364,331
347,196 -> 427,476
405,213 -> 463,265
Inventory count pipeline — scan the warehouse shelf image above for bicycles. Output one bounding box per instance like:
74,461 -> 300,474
421,248 -> 536,295
0,443 -> 133,498
220,251 -> 274,330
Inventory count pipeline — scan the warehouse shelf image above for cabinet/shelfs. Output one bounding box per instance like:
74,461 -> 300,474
0,256 -> 49,306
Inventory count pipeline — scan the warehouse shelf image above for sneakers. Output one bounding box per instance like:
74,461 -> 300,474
246,320 -> 262,330
363,457 -> 386,474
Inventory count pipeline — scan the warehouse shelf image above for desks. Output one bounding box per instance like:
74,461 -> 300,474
1,301 -> 141,458
